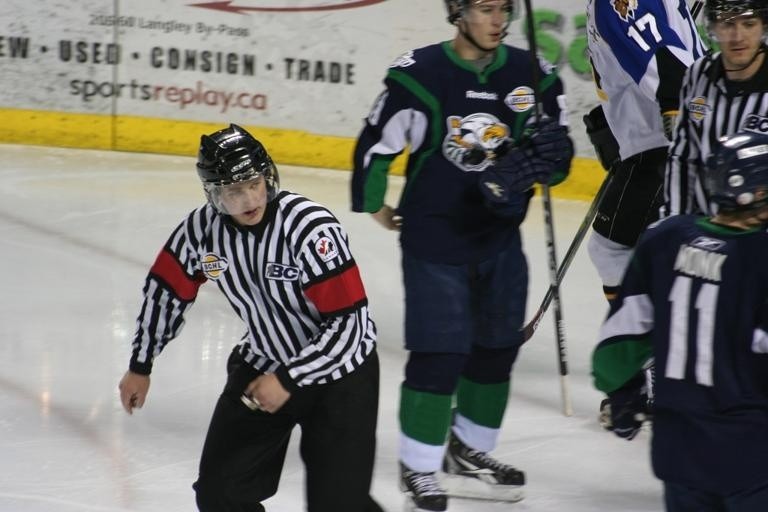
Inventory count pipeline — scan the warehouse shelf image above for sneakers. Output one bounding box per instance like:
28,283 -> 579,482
396,459 -> 448,511
441,429 -> 525,486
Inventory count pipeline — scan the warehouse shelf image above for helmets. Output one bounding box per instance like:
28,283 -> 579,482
701,130 -> 767,217
442,0 -> 523,28
193,120 -> 281,217
699,0 -> 767,29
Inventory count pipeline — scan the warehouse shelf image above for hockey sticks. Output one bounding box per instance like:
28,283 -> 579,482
520,0 -> 704,343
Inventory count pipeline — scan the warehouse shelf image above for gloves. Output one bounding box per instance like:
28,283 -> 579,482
473,116 -> 575,207
598,370 -> 655,442
579,103 -> 622,171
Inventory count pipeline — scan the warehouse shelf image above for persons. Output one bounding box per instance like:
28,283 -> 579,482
118,123 -> 386,511
349,0 -> 575,512
583,0 -> 706,312
590,134 -> 766,512
656,0 -> 767,219
